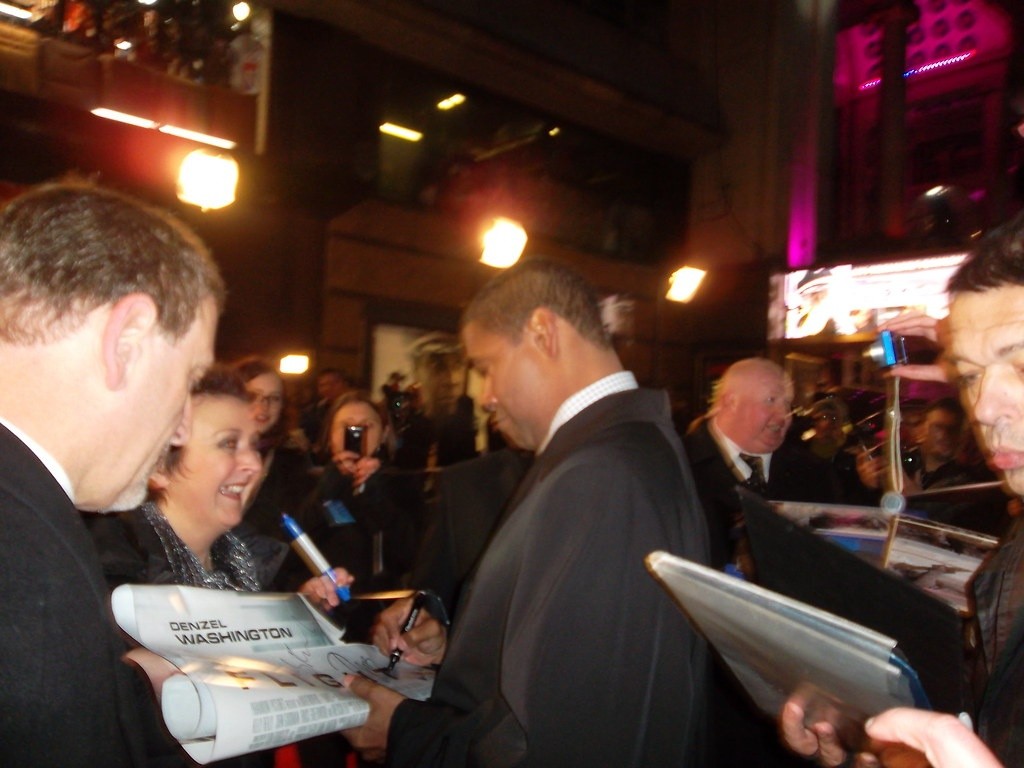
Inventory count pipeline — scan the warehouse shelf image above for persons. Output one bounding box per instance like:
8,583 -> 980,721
2,172 -> 1024,767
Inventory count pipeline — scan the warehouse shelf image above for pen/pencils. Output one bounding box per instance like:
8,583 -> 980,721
957,710 -> 975,733
281,511 -> 350,601
387,592 -> 425,670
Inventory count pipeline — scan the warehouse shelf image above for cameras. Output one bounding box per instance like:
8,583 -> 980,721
860,330 -> 907,373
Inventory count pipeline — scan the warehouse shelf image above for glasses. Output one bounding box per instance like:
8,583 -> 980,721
244,387 -> 286,409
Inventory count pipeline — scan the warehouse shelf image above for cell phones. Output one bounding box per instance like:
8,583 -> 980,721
900,446 -> 926,481
343,423 -> 367,457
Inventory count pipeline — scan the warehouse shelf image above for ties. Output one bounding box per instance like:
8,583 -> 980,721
739,452 -> 770,499
